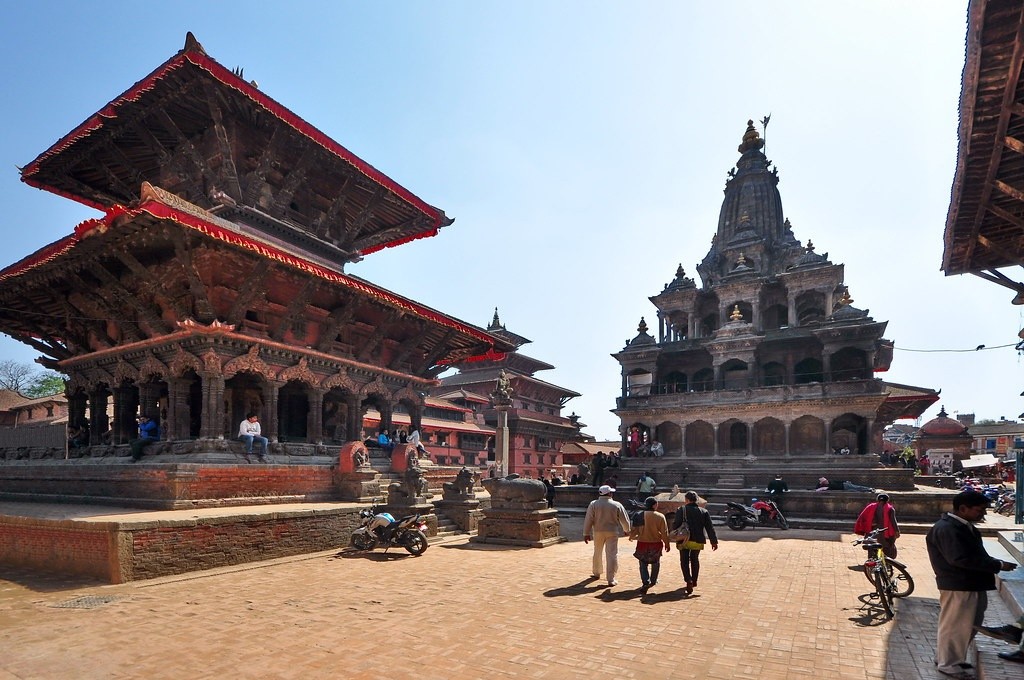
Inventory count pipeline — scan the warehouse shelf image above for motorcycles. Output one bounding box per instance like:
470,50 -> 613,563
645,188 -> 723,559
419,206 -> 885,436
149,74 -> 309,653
350,498 -> 429,556
953,472 -> 1016,517
725,487 -> 789,531
625,499 -> 648,527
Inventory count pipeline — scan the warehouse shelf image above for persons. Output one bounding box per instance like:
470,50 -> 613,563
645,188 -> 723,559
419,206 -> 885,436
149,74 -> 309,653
926,491 -> 1017,680
582,484 -> 631,586
883,450 -> 930,474
101,422 -> 113,445
129,414 -> 160,463
958,463 -> 1016,482
672,490 -> 718,594
406,424 -> 431,455
832,445 -> 850,455
493,368 -> 514,398
237,412 -> 269,458
765,473 -> 789,520
971,612 -> 1024,666
629,496 -> 670,588
816,476 -> 876,493
377,429 -> 407,462
70,420 -> 90,449
542,426 -> 665,508
853,493 -> 901,561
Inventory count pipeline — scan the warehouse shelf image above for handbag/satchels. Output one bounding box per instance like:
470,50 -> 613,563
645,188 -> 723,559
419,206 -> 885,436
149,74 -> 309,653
669,505 -> 690,541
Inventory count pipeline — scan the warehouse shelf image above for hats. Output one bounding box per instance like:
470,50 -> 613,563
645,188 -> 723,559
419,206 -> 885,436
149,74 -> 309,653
599,484 -> 615,496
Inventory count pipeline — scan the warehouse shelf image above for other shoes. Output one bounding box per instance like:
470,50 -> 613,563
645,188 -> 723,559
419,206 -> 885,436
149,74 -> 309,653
693,578 -> 697,586
643,580 -> 649,585
595,574 -> 601,578
938,668 -> 976,680
974,624 -> 1023,644
998,650 -> 1024,663
651,580 -> 656,584
609,580 -> 618,586
934,660 -> 974,669
686,580 -> 693,594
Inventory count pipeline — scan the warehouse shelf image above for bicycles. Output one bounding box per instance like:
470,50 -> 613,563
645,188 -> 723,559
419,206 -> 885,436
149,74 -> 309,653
850,526 -> 915,618
